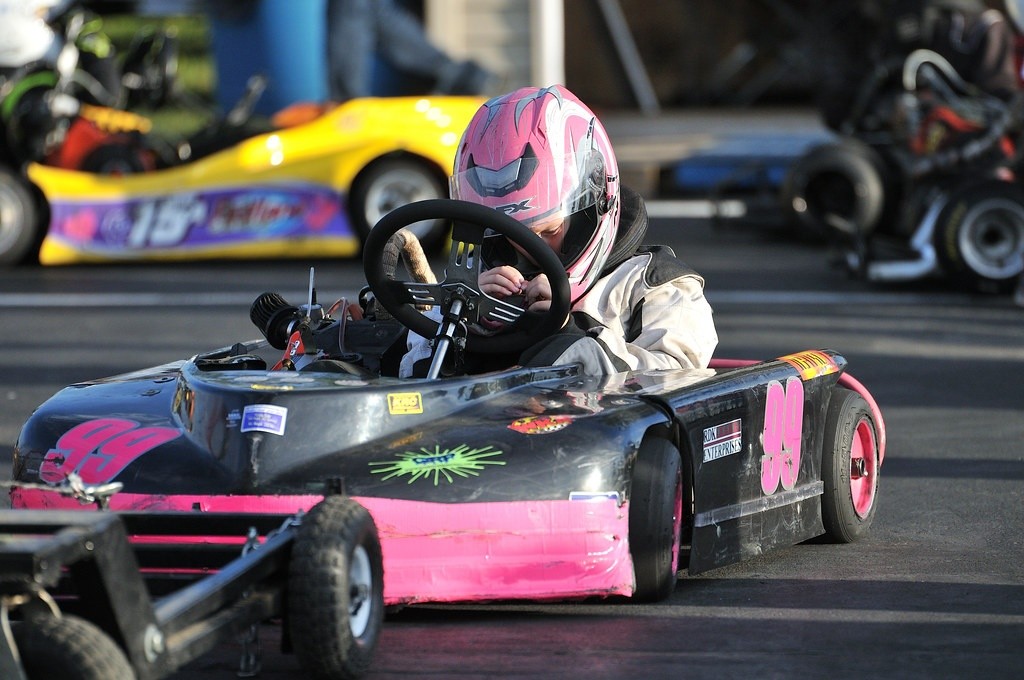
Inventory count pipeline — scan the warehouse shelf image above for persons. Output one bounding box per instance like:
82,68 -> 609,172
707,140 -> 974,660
0,1 -> 530,173
397,86 -> 720,392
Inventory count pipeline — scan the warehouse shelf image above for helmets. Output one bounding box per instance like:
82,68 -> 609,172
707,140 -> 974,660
448,84 -> 621,311
